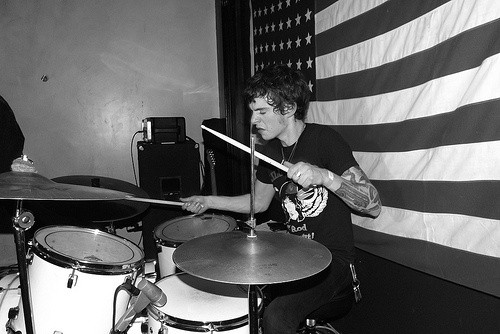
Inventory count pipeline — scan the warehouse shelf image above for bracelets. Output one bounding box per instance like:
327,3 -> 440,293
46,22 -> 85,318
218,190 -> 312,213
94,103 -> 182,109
326,171 -> 334,187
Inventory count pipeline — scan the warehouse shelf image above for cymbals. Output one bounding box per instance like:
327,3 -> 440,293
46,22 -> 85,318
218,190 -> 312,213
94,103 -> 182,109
49,175 -> 151,222
172,230 -> 333,285
0,171 -> 135,201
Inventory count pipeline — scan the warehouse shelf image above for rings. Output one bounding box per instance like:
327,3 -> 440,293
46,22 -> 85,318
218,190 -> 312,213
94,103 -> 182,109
295,172 -> 302,178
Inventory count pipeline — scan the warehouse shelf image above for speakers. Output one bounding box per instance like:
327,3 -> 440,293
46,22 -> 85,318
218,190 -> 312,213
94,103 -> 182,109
139,140 -> 201,260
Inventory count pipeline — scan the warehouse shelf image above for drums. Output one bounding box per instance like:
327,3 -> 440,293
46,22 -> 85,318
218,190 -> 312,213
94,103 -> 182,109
0,225 -> 145,334
152,213 -> 240,280
146,271 -> 266,334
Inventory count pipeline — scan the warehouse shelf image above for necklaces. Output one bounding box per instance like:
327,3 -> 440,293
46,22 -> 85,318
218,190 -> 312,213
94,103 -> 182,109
281,125 -> 305,165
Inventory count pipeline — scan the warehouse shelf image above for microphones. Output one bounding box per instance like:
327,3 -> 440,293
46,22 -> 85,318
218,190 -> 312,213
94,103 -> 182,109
137,280 -> 167,306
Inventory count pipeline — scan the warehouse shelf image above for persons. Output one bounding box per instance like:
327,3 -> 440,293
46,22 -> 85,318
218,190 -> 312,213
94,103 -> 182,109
180,64 -> 381,334
0,95 -> 25,172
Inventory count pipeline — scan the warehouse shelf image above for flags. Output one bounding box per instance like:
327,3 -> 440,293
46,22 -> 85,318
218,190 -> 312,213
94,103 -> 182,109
250,0 -> 500,297
